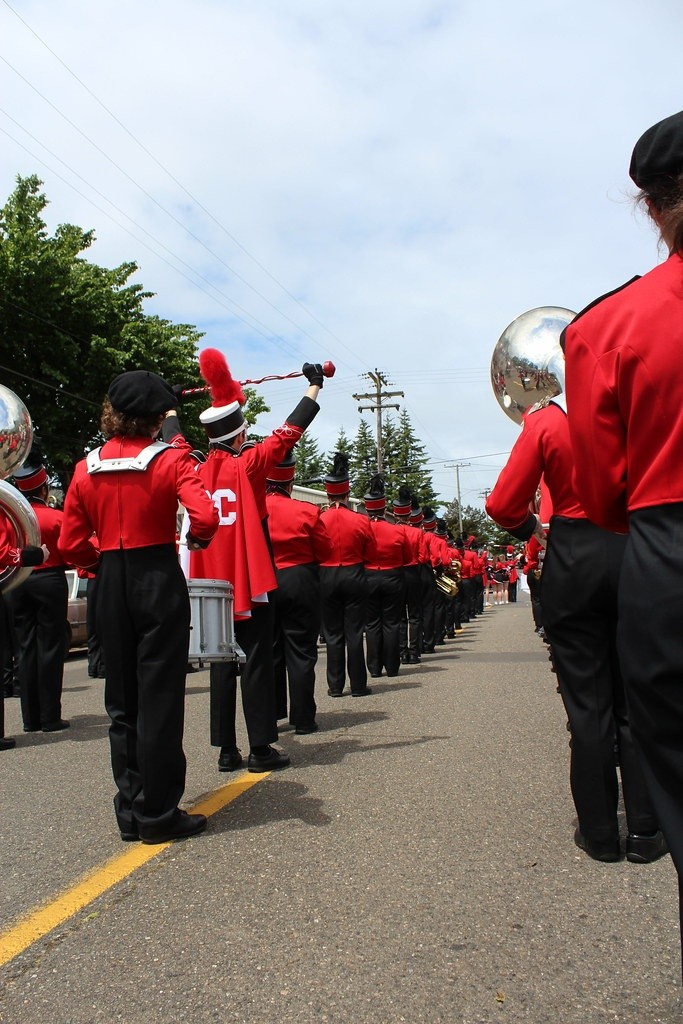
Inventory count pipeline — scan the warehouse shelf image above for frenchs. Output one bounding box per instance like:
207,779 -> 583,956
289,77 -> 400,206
0,383 -> 41,595
488,306 -> 578,581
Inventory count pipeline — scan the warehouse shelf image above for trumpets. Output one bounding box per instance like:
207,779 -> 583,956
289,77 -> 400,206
451,559 -> 462,571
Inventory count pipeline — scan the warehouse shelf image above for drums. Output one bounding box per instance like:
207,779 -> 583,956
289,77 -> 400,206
185,578 -> 237,663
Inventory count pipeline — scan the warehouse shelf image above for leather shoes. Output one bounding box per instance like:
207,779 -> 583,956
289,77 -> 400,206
400,608 -> 484,664
625,831 -> 669,863
120,805 -> 208,844
218,748 -> 242,771
249,745 -> 290,772
574,827 -> 621,861
294,722 -> 318,735
369,670 -> 400,678
0,737 -> 16,751
22,720 -> 70,732
534,625 -> 621,768
351,686 -> 372,697
327,689 -> 343,697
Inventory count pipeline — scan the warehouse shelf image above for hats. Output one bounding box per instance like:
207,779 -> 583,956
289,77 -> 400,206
108,370 -> 178,417
448,529 -> 479,556
364,475 -> 386,512
265,443 -> 296,486
324,453 -> 351,496
629,111 -> 683,188
199,349 -> 248,444
393,485 -> 447,537
12,442 -> 48,493
507,545 -> 514,559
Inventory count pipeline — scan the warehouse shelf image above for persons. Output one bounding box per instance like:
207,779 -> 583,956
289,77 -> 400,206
559,110 -> 683,966
0,348 -> 669,865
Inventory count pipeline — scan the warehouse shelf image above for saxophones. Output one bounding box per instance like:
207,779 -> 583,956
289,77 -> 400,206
433,569 -> 458,597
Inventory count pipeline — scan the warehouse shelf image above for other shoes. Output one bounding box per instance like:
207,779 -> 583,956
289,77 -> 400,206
486,600 -> 511,606
